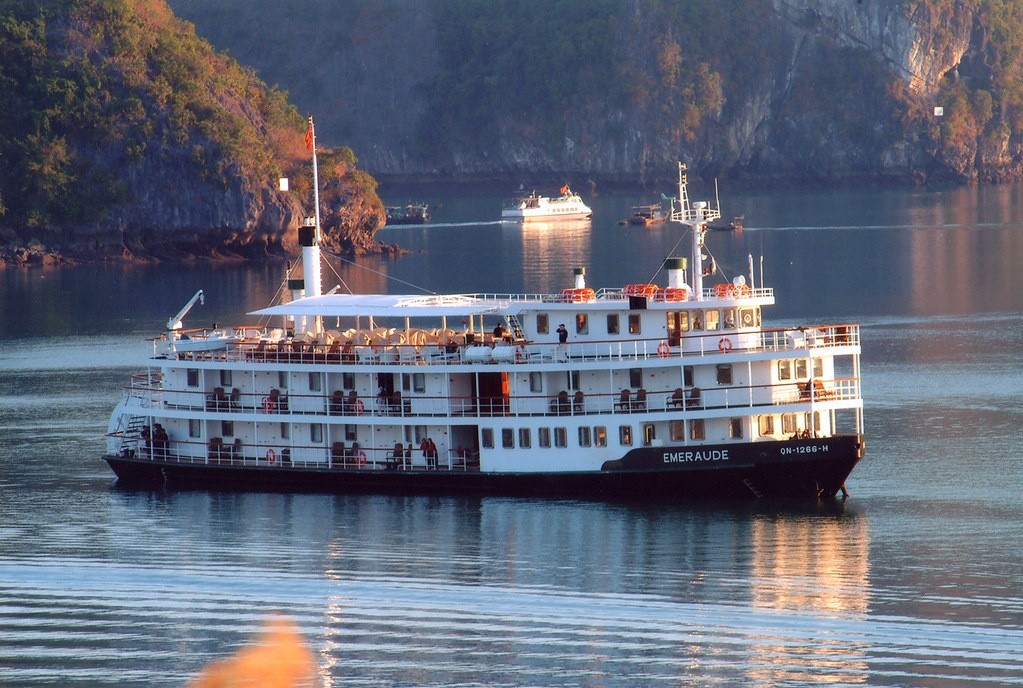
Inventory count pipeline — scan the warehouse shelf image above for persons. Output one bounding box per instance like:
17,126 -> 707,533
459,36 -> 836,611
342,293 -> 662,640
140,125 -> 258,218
419,438 -> 439,470
555,323 -> 569,363
693,318 -> 704,331
376,384 -> 386,416
141,423 -> 171,458
724,316 -> 735,328
492,323 -> 505,343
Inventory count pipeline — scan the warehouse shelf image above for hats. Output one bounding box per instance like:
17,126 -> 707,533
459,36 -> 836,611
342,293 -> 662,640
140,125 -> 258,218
559,323 -> 565,327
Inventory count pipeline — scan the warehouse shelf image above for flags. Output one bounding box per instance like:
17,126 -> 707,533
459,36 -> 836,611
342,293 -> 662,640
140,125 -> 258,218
304,126 -> 313,149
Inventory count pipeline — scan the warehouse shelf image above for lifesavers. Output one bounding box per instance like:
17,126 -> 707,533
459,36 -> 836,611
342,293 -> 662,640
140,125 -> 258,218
357,452 -> 366,467
266,449 -> 275,464
354,400 -> 364,415
744,314 -> 752,324
718,338 -> 732,353
726,315 -> 734,324
657,342 -> 670,357
263,399 -> 273,413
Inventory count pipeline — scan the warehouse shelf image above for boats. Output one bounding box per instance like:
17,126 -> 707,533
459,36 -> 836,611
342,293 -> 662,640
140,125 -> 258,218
501,182 -> 593,222
629,205 -> 665,226
384,206 -> 402,225
402,201 -> 432,224
102,114 -> 864,489
